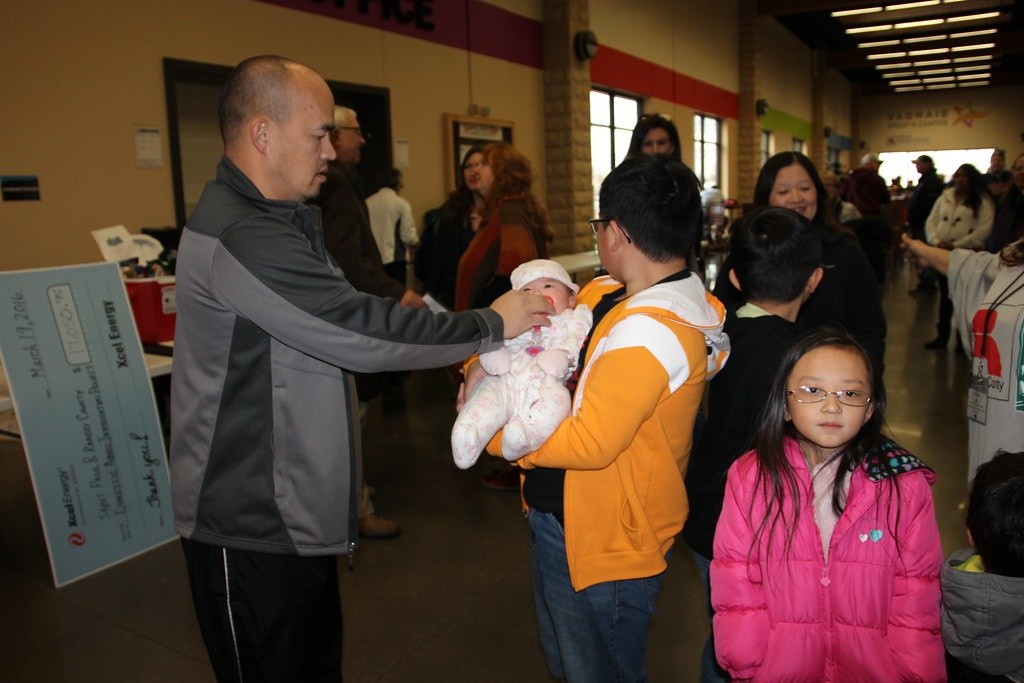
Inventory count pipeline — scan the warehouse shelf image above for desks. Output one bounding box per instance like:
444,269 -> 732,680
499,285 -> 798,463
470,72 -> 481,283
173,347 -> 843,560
1,345 -> 178,437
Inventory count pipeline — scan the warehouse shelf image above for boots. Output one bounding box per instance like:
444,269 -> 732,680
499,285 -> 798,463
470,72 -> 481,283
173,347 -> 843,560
924,323 -> 949,349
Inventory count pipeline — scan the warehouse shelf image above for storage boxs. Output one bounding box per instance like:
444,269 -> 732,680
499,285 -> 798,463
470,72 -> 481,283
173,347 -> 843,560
125,278 -> 176,343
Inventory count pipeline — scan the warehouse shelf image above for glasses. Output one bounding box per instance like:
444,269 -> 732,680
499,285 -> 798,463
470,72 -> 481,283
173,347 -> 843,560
340,125 -> 367,137
590,217 -> 634,244
780,386 -> 873,409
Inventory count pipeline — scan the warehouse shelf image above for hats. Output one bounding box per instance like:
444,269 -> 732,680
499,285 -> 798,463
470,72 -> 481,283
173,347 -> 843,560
510,257 -> 581,298
861,152 -> 884,166
911,154 -> 932,164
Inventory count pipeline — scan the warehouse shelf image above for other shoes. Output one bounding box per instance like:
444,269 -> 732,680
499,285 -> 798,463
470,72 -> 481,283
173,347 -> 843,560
357,511 -> 398,538
916,281 -> 936,291
484,466 -> 524,490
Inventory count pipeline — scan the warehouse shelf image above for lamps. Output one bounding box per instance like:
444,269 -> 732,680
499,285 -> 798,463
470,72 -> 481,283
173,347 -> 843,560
861,140 -> 867,150
824,127 -> 832,137
755,97 -> 769,116
573,31 -> 599,60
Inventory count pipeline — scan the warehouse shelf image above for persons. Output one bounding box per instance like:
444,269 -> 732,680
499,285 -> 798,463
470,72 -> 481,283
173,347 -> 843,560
171,55 -> 556,683
683,204 -> 824,683
710,330 -> 946,683
457,155 -> 731,683
840,153 -> 893,285
456,141 -> 555,312
752,151 -> 886,385
899,233 -> 1024,485
908,155 -> 944,298
820,169 -> 865,232
699,185 -> 728,242
625,114 -> 683,159
984,149 -> 1024,252
413,146 -> 484,308
924,164 -> 993,350
367,167 -> 418,284
304,105 -> 428,539
452,259 -> 593,470
939,451 -> 1024,683
